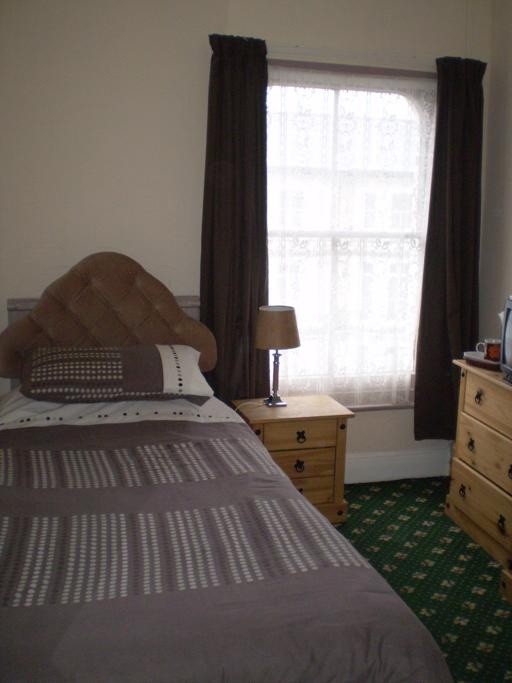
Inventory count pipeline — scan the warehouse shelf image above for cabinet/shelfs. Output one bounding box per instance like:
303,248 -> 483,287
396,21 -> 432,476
444,359 -> 512,575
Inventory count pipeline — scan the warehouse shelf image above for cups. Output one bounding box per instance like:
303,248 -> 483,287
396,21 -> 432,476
476,338 -> 502,363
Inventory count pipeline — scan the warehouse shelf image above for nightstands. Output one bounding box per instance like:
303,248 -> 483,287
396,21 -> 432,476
232,394 -> 356,528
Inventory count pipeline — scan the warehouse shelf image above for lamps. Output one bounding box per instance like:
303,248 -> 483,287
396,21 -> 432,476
253,305 -> 300,407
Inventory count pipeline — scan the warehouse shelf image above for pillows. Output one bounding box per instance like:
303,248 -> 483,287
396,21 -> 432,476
20,345 -> 213,400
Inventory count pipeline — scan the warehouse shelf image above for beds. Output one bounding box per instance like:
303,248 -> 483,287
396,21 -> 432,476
0,253 -> 451,683
